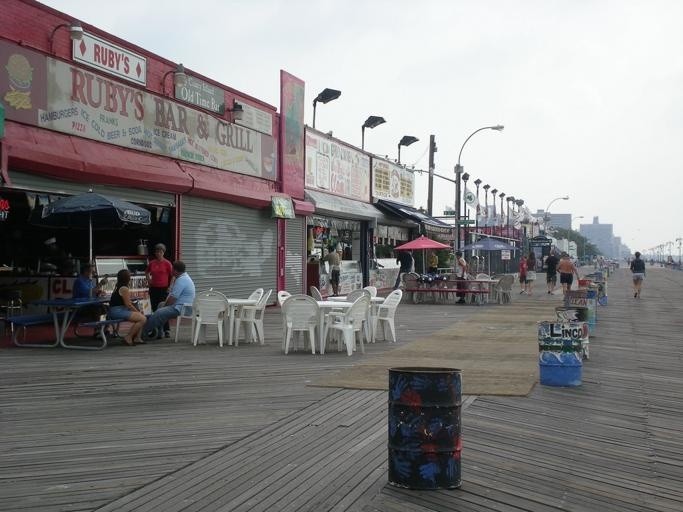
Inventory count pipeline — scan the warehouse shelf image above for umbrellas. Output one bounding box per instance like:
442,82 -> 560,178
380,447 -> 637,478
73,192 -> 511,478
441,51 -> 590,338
393,234 -> 453,274
27,188 -> 151,297
458,235 -> 520,277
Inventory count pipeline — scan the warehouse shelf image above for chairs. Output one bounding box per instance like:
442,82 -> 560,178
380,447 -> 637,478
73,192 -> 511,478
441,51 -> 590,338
241,287 -> 263,343
174,287 -> 214,345
399,271 -> 421,304
361,286 -> 377,299
323,294 -> 371,357
310,285 -> 323,301
276,290 -> 292,306
281,293 -> 322,355
347,289 -> 371,306
235,287 -> 272,347
372,289 -> 403,343
475,273 -> 491,281
493,274 -> 515,305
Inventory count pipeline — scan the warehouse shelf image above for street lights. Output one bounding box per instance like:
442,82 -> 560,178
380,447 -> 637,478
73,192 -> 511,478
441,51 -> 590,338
399,136 -> 420,164
676,238 -> 683,264
492,189 -> 498,236
568,216 -> 584,256
544,197 -> 569,236
666,241 -> 674,256
474,179 -> 481,243
462,173 -> 469,261
483,184 -> 490,238
454,126 -> 505,273
499,192 -> 505,242
507,197 -> 512,238
649,244 -> 667,262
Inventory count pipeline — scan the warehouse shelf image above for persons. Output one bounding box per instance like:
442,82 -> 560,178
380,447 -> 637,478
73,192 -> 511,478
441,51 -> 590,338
146,244 -> 175,339
546,249 -> 559,296
392,248 -> 412,291
599,257 -> 603,269
631,251 -> 646,299
525,252 -> 536,296
321,246 -> 342,297
427,250 -> 438,276
519,255 -> 528,295
556,254 -> 580,295
456,250 -> 467,304
142,260 -> 195,341
593,255 -> 599,270
73,263 -> 108,337
109,269 -> 147,345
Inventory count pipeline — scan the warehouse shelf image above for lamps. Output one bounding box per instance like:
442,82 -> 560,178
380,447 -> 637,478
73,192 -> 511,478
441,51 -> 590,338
46,19 -> 84,55
397,134 -> 420,168
156,64 -> 186,101
218,98 -> 245,127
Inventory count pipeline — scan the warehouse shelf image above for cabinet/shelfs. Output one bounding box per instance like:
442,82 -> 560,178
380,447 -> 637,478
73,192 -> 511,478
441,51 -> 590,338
92,255 -> 152,317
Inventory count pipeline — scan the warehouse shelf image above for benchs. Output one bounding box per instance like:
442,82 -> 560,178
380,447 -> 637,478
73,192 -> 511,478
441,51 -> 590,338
405,287 -> 489,305
82,317 -> 126,349
9,310 -> 80,348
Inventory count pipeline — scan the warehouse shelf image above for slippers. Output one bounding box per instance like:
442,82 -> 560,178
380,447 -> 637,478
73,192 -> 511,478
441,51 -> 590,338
134,341 -> 147,344
123,337 -> 137,346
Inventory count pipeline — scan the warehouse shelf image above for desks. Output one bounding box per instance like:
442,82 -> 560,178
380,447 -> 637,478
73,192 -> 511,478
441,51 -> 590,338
227,299 -> 257,346
325,296 -> 386,315
406,278 -> 500,286
31,295 -> 144,350
316,302 -> 354,308
